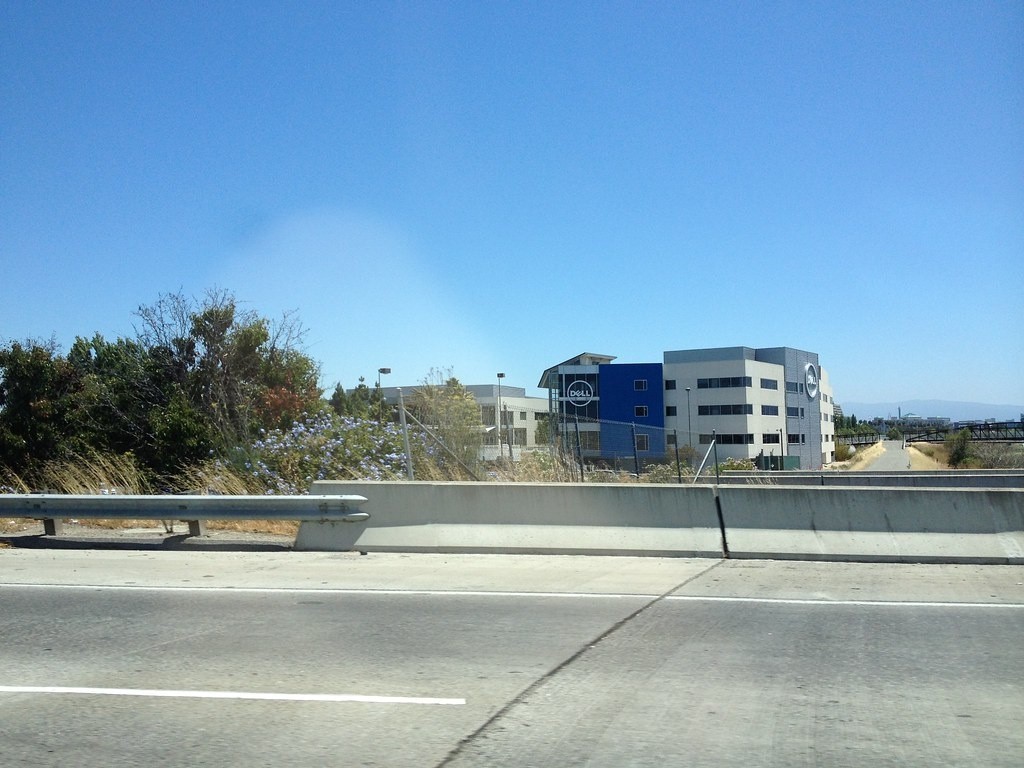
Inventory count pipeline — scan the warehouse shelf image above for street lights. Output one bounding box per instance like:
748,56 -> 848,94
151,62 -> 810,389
684,387 -> 692,447
497,372 -> 506,457
378,367 -> 392,421
